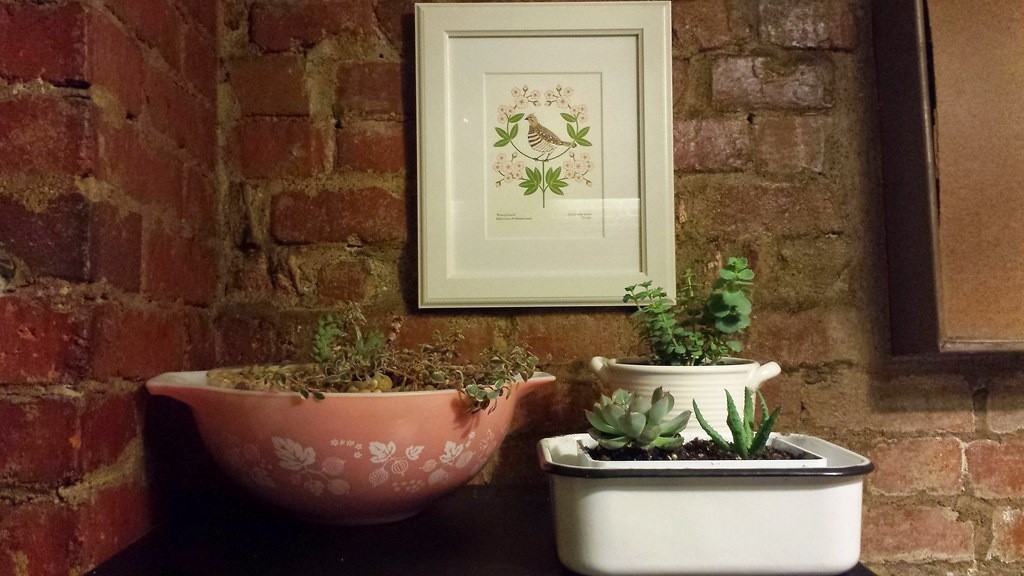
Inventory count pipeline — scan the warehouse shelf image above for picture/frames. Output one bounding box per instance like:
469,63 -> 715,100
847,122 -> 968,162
413,2 -> 677,310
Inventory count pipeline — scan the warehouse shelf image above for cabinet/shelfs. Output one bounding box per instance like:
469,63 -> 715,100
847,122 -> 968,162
871,0 -> 1024,357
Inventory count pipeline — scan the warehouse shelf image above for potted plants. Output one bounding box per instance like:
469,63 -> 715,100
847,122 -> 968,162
591,255 -> 782,445
144,303 -> 557,528
535,431 -> 874,576
